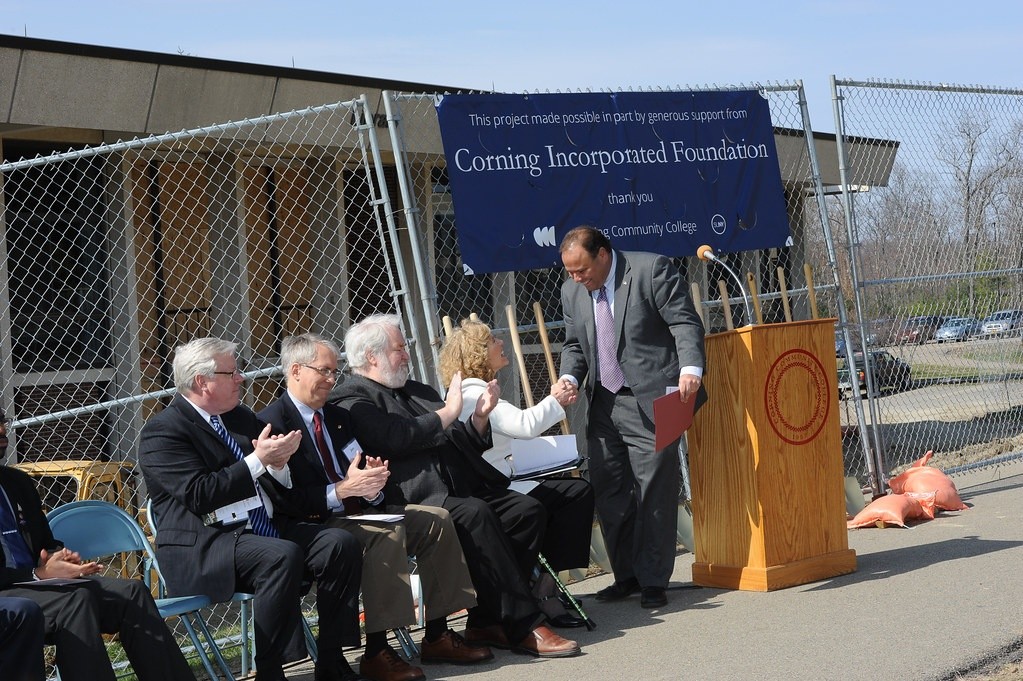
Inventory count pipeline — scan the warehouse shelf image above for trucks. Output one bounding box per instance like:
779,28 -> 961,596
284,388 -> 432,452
7,459 -> 192,643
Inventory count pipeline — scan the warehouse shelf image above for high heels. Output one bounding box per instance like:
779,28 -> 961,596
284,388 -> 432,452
558,593 -> 582,607
533,594 -> 586,626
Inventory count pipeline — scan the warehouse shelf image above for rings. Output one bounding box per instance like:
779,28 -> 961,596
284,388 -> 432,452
80,573 -> 84,577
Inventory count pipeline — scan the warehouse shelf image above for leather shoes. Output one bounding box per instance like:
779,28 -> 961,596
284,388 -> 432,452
510,624 -> 580,657
421,628 -> 494,664
464,625 -> 515,649
596,583 -> 639,602
359,644 -> 426,681
641,586 -> 668,608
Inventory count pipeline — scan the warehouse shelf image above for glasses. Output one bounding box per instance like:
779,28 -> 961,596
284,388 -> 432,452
299,363 -> 341,379
211,368 -> 240,380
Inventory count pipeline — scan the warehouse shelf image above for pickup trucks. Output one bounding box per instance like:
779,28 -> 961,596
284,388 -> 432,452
837,351 -> 912,400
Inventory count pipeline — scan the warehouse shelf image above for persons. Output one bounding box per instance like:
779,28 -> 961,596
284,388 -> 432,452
328,314 -> 582,655
438,319 -> 595,627
140,339 -> 363,680
556,227 -> 705,606
255,334 -> 495,681
0,410 -> 194,680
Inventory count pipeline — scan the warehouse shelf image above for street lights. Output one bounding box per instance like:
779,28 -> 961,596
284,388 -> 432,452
991,221 -> 1001,310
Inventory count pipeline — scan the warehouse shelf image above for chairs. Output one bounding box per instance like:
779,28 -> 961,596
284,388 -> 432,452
43,496 -> 426,681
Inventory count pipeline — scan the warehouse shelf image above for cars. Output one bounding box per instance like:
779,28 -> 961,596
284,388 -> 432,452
824,310 -> 1023,357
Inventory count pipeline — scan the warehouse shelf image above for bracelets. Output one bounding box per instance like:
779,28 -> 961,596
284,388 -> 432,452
363,492 -> 381,501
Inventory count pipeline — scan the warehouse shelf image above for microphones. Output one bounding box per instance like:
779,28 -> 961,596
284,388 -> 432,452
697,244 -> 756,327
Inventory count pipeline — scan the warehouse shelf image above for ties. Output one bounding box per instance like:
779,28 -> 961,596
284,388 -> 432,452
313,411 -> 351,514
210,414 -> 279,539
0,486 -> 32,570
393,389 -> 458,492
596,285 -> 625,394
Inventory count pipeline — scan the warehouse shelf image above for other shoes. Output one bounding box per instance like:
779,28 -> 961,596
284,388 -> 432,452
314,656 -> 364,681
254,671 -> 288,681
